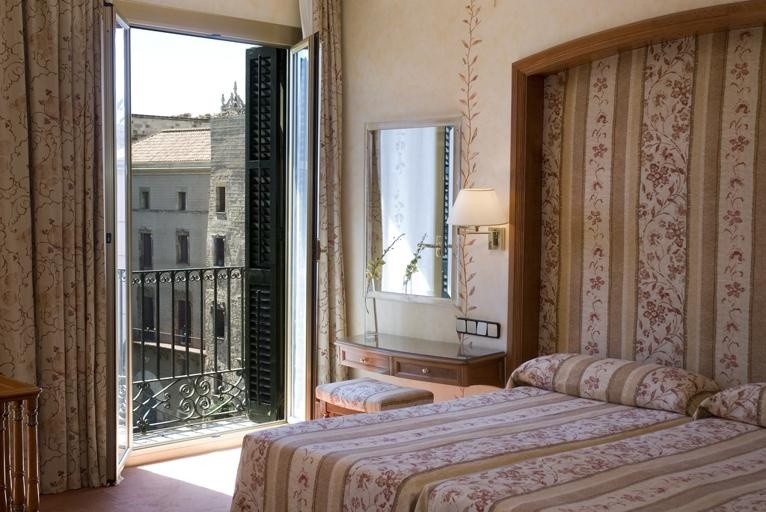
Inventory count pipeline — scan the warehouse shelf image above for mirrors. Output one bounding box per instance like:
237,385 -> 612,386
365,114 -> 461,307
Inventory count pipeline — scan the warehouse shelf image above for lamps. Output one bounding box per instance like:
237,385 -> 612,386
446,189 -> 509,250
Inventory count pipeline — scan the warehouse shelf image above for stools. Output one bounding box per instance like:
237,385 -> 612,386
314,378 -> 433,420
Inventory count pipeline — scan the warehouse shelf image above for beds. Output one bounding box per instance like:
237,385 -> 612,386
247,387 -> 764,512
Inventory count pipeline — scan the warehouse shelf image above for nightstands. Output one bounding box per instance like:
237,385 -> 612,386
335,332 -> 504,396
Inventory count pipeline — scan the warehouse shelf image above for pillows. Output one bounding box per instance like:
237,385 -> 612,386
506,353 -> 763,429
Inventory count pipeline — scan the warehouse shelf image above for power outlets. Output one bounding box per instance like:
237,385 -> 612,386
456,319 -> 499,339
